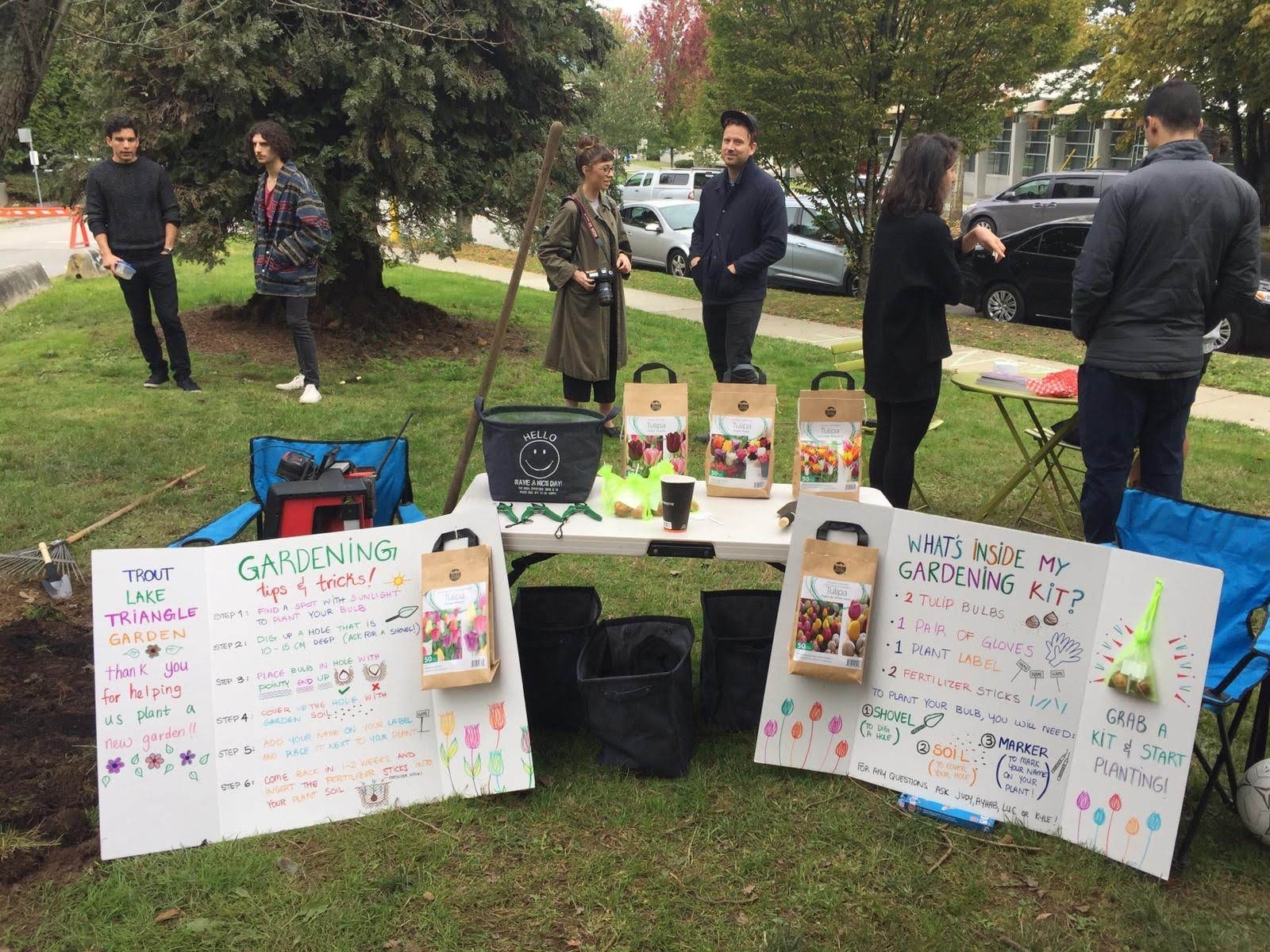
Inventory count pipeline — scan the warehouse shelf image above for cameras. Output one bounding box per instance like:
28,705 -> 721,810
585,267 -> 616,306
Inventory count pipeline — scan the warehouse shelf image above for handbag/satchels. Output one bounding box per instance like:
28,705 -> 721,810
705,362 -> 778,499
792,371 -> 865,503
575,611 -> 695,776
472,395 -> 622,504
693,588 -> 782,733
789,520 -> 880,687
418,527 -> 501,691
1027,367 -> 1080,398
542,196 -> 581,291
621,362 -> 689,491
513,584 -> 603,728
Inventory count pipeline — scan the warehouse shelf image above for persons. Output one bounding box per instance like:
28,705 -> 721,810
863,133 -> 1006,510
83,115 -> 201,393
245,119 -> 331,404
686,110 -> 788,446
1069,80 -> 1262,544
536,134 -> 633,437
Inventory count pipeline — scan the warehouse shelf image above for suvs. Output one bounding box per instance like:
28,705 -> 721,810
960,169 -> 1131,247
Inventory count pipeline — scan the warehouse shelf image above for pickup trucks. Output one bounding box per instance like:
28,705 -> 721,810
607,167 -> 724,205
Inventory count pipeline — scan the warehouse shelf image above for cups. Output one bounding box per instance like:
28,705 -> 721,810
660,474 -> 696,533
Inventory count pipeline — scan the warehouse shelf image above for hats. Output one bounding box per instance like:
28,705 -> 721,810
721,110 -> 758,140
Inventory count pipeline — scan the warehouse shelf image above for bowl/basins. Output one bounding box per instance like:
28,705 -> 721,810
115,260 -> 139,280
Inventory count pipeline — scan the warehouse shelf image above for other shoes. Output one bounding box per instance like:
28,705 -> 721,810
602,421 -> 624,439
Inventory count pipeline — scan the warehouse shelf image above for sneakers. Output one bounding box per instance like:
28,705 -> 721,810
299,383 -> 322,403
276,372 -> 305,390
143,372 -> 170,388
177,378 -> 202,393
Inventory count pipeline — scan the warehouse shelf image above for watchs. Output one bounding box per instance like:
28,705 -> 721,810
163,247 -> 174,255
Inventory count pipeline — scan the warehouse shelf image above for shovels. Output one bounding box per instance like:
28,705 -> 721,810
39,541 -> 72,600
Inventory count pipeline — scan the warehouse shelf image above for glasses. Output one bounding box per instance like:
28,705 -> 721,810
596,165 -> 616,175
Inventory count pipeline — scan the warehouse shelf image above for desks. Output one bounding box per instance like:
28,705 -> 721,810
951,369 -> 1090,543
446,471 -> 895,588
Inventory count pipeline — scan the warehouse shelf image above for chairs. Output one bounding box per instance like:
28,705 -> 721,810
830,337 -> 943,517
1093,485 -> 1270,882
160,437 -> 428,554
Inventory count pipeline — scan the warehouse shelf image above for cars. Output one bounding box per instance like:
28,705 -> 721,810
766,176 -> 888,297
617,200 -> 700,277
960,215 -> 1270,356
